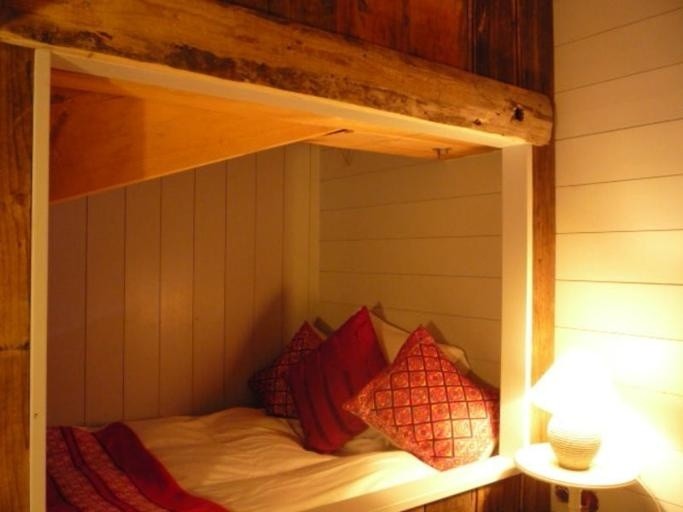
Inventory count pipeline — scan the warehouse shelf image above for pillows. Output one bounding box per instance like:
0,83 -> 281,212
246,318 -> 328,419
354,307 -> 470,377
343,324 -> 500,471
281,307 -> 390,454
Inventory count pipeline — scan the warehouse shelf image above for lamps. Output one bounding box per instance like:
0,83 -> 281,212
531,361 -> 614,471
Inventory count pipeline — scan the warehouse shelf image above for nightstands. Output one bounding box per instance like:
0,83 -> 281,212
516,441 -> 639,512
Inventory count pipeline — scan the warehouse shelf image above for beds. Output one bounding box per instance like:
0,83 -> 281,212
0,0 -> 553,511
